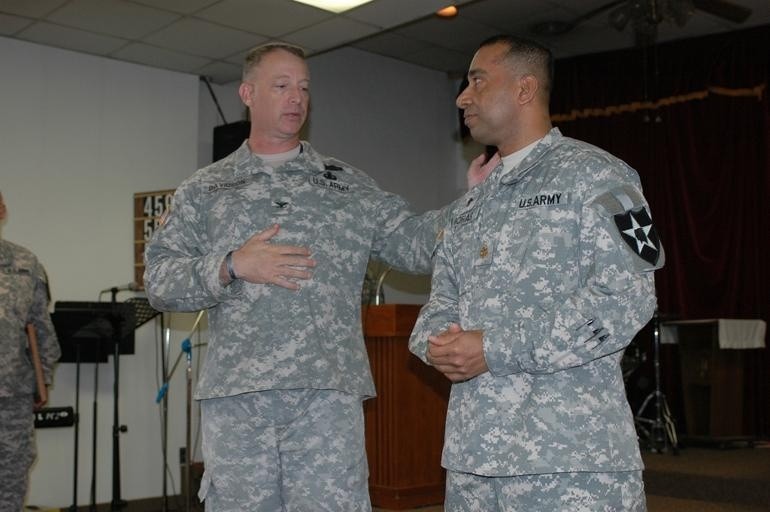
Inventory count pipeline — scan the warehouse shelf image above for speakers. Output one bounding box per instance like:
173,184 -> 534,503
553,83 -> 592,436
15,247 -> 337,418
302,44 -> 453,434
212,119 -> 250,162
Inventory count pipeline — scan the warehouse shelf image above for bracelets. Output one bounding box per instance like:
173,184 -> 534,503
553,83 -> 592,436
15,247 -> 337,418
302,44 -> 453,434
225,250 -> 238,281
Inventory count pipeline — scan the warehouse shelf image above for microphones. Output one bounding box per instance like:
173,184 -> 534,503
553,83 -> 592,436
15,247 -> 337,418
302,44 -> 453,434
101,282 -> 140,294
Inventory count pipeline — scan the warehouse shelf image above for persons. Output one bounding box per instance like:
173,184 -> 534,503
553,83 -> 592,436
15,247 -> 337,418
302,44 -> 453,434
408,32 -> 672,509
0,196 -> 64,511
143,44 -> 503,512
